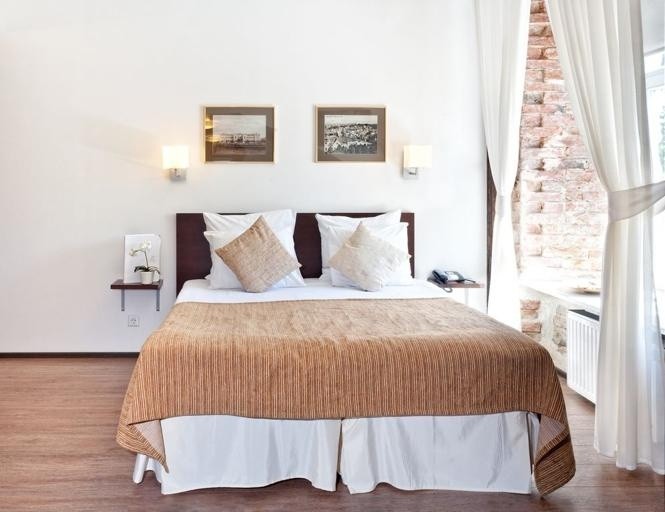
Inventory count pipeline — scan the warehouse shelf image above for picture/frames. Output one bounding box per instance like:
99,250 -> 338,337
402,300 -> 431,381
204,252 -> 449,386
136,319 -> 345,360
201,104 -> 277,165
315,104 -> 389,164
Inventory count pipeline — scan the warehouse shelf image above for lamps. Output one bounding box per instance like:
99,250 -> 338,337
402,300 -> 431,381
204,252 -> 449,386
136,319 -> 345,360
163,144 -> 189,176
403,144 -> 432,179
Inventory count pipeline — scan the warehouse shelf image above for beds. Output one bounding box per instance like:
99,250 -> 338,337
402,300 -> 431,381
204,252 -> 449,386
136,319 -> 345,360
116,212 -> 577,498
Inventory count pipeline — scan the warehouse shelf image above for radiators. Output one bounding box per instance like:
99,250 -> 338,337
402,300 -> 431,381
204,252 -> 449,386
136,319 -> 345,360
567,308 -> 600,406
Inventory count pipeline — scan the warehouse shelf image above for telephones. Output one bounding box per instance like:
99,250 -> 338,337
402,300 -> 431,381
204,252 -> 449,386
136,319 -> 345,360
432,269 -> 465,286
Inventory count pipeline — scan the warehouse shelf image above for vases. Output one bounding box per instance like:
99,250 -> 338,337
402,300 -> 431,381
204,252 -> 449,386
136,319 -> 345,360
140,270 -> 153,285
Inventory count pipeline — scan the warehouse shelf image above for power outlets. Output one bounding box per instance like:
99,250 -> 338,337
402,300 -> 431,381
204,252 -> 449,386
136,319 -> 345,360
128,314 -> 139,326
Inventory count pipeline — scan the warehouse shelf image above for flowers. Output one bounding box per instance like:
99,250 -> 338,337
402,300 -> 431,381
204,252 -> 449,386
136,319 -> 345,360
129,239 -> 160,276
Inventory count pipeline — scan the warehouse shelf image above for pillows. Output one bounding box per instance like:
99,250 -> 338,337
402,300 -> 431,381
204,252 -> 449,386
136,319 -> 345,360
202,209 -> 306,293
314,209 -> 413,292
